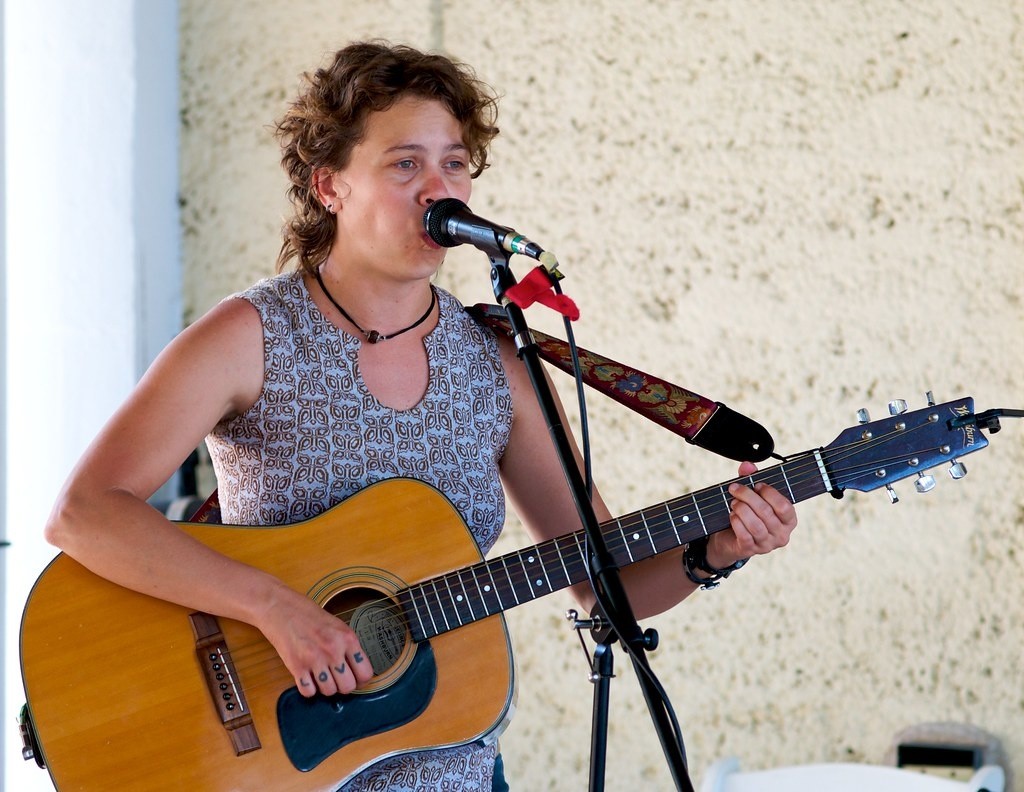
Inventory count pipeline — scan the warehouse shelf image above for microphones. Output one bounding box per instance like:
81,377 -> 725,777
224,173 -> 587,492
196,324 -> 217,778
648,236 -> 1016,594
423,197 -> 545,259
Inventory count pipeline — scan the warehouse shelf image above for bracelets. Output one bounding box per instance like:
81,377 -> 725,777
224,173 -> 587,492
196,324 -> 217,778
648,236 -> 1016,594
682,534 -> 748,588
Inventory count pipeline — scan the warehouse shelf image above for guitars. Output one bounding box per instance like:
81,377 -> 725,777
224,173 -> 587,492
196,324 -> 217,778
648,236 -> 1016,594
17,389 -> 993,792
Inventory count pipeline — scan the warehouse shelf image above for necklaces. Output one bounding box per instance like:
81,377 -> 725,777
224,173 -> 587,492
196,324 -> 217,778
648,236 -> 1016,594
314,261 -> 435,343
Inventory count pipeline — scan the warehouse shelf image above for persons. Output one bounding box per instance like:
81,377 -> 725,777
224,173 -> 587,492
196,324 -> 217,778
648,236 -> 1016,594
44,43 -> 797,792
